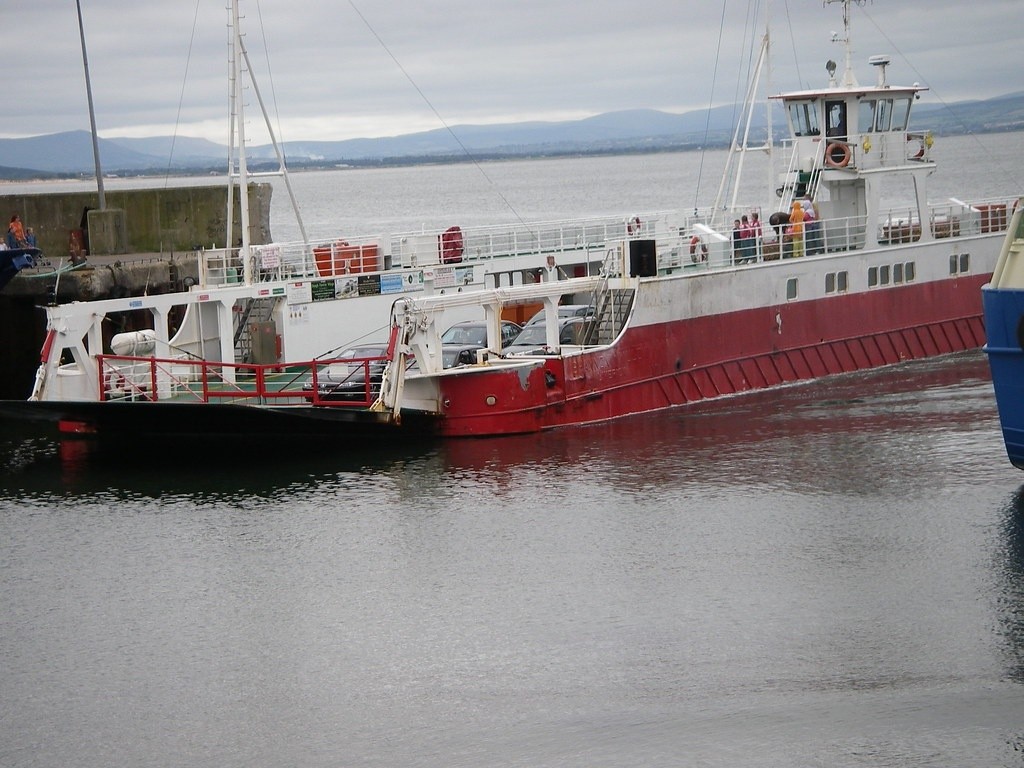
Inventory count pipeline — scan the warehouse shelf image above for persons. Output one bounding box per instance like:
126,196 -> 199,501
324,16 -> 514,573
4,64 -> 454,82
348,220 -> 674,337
768,211 -> 790,239
789,193 -> 823,257
0,237 -> 8,250
25,227 -> 36,249
8,214 -> 31,248
729,212 -> 763,264
838,113 -> 845,128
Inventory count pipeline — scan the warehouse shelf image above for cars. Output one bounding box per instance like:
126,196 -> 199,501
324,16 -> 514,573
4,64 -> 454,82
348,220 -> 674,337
441,318 -> 525,370
301,342 -> 411,407
522,304 -> 596,333
499,315 -> 597,360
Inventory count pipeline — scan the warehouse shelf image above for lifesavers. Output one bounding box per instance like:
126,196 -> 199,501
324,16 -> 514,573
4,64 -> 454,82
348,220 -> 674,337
627,216 -> 641,234
690,237 -> 706,263
826,143 -> 850,167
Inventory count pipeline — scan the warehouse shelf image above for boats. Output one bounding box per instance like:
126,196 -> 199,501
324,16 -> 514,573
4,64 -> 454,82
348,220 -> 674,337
980,194 -> 1024,472
0,0 -> 1024,444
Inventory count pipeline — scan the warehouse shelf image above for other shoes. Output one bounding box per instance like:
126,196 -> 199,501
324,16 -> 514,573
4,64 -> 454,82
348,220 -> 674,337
738,260 -> 746,265
747,259 -> 753,264
